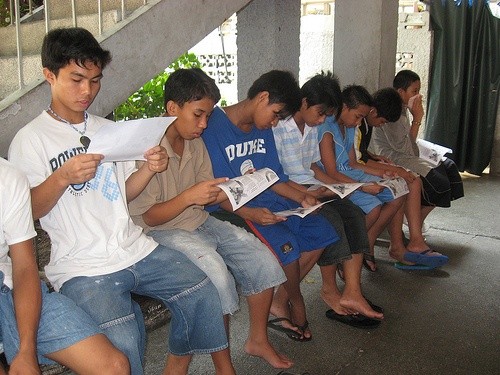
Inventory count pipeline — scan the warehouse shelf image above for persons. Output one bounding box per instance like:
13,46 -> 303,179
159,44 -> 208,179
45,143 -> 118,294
125,67 -> 296,375
371,71 -> 464,225
317,85 -> 411,281
7,27 -> 229,375
272,73 -> 384,328
0,157 -> 131,375
199,70 -> 341,341
354,87 -> 449,270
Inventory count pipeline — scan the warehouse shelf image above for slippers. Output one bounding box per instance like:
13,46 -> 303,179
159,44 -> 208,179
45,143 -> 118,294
362,253 -> 378,273
267,317 -> 301,338
394,261 -> 433,270
286,320 -> 313,341
403,248 -> 448,267
325,309 -> 381,329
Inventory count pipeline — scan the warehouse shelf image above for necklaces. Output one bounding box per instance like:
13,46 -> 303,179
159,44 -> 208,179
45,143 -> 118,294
47,106 -> 91,149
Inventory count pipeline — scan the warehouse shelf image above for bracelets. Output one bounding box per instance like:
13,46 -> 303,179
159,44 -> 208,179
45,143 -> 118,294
412,121 -> 421,125
